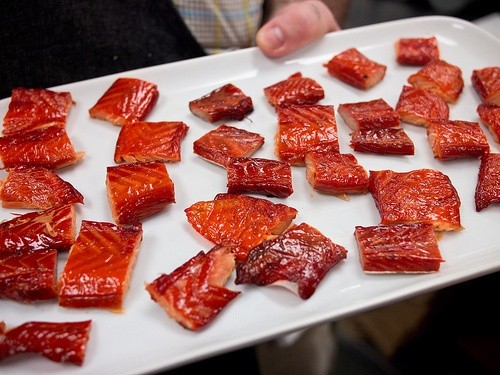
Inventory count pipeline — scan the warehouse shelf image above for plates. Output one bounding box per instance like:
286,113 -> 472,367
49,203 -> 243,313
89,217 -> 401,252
1,14 -> 499,375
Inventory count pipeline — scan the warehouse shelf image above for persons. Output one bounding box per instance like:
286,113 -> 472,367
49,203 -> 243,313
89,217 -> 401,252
3,0 -> 347,375
